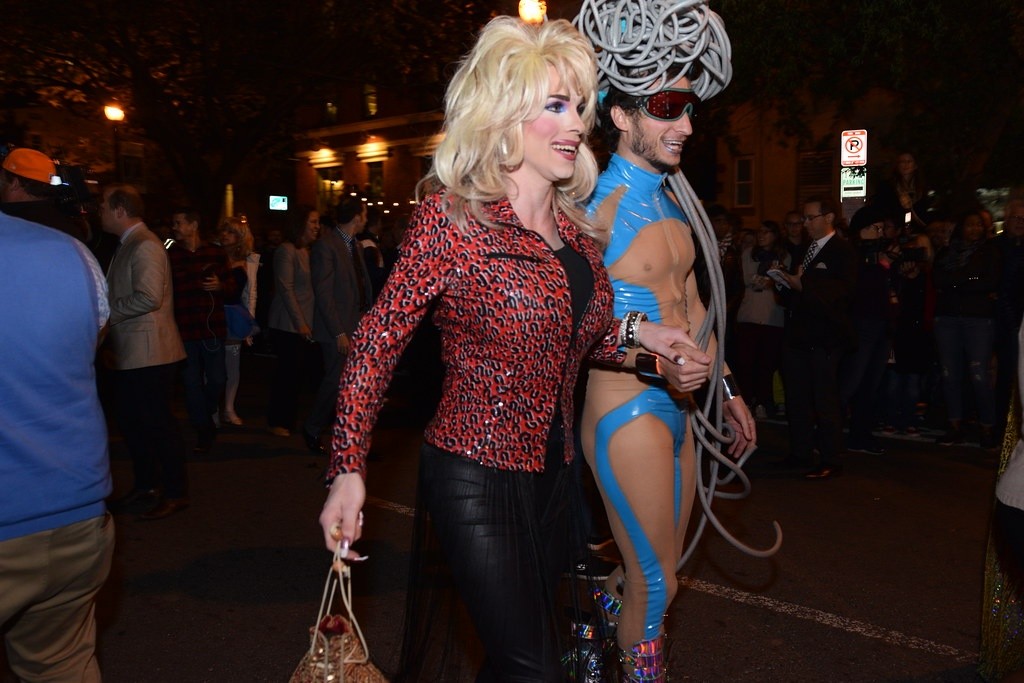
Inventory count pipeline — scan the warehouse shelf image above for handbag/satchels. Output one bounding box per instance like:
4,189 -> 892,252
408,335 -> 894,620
288,529 -> 385,683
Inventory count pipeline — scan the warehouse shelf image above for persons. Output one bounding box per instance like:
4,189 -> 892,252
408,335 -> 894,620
562,1 -> 754,683
707,146 -> 1024,484
317,13 -> 702,683
990,318 -> 1024,581
1,213 -> 115,683
0,147 -> 440,523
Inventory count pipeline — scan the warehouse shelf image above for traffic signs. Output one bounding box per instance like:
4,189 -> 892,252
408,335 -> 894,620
840,130 -> 867,167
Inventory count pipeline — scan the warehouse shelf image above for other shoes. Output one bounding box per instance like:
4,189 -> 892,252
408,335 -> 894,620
276,426 -> 292,437
805,465 -> 832,478
880,425 -> 897,436
303,431 -> 329,455
110,489 -> 150,516
138,496 -> 191,520
906,426 -> 920,438
223,409 -> 242,427
849,439 -> 886,455
782,455 -> 814,472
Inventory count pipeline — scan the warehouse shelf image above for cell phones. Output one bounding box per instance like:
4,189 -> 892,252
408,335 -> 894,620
767,269 -> 785,282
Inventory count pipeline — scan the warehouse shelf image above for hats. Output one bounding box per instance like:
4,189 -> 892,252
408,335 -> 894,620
1,147 -> 58,183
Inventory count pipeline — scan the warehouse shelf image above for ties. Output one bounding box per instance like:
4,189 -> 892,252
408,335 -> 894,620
350,239 -> 366,297
799,241 -> 819,273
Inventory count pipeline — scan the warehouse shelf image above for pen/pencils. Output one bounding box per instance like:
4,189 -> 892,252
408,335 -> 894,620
778,266 -> 787,269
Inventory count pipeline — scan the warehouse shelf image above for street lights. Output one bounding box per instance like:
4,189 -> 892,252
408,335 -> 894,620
101,96 -> 127,185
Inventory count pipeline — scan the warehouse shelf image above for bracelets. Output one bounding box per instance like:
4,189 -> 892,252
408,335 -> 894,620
619,311 -> 649,348
721,374 -> 741,403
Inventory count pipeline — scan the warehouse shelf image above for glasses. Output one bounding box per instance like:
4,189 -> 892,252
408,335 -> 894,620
624,87 -> 698,120
802,212 -> 832,222
